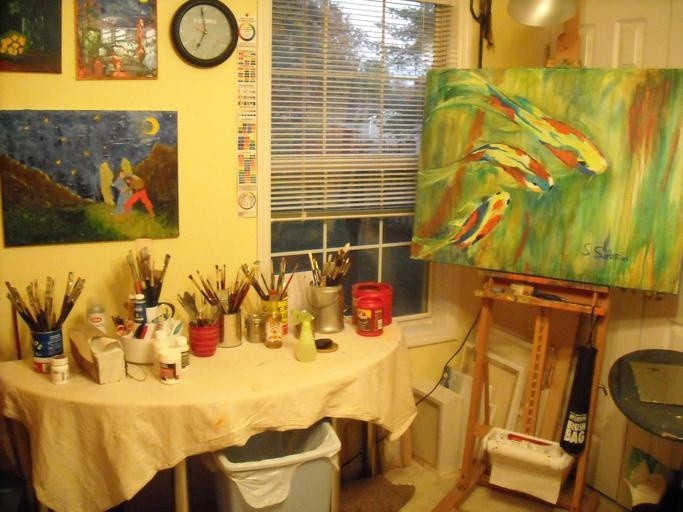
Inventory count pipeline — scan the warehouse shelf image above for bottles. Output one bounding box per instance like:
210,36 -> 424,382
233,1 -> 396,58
176,336 -> 190,367
50,355 -> 70,385
264,289 -> 282,349
88,299 -> 106,335
128,293 -> 146,322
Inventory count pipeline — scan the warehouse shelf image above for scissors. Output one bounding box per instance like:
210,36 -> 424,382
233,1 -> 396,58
116,320 -> 134,336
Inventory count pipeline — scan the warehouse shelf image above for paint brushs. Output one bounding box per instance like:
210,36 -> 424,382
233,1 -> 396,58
5,272 -> 85,332
307,242 -> 352,287
178,257 -> 299,327
126,247 -> 170,308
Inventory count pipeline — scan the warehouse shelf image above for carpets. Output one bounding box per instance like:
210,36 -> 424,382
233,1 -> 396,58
340,473 -> 415,512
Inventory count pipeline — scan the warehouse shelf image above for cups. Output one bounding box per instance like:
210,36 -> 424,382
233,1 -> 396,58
159,349 -> 182,385
213,311 -> 242,348
145,302 -> 175,323
155,330 -> 176,356
305,282 -> 343,333
30,332 -> 65,373
261,301 -> 288,334
188,320 -> 219,357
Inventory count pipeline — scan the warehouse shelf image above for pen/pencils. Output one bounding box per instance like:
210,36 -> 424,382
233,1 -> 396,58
134,321 -> 147,339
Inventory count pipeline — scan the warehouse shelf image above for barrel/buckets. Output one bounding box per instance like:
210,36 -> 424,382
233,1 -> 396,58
351,282 -> 394,328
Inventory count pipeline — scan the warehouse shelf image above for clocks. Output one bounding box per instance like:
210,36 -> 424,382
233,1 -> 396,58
170,0 -> 238,66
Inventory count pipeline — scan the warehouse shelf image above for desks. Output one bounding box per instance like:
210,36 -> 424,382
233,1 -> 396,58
0,316 -> 416,512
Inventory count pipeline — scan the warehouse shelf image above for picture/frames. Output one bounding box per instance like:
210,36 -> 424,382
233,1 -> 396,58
411,323 -> 557,477
